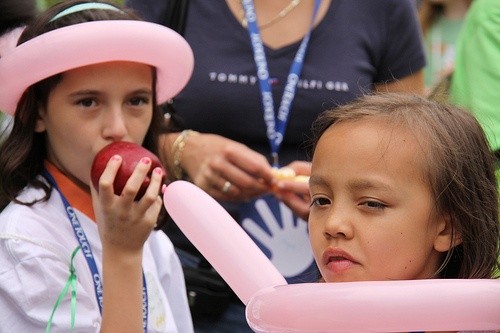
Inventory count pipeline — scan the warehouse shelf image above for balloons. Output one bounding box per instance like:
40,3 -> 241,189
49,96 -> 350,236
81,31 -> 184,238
0,20 -> 195,116
163,181 -> 500,333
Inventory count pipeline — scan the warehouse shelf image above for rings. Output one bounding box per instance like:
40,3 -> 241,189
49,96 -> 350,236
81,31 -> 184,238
222,181 -> 232,193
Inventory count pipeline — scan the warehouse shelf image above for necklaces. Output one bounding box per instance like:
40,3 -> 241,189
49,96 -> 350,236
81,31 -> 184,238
241,0 -> 301,30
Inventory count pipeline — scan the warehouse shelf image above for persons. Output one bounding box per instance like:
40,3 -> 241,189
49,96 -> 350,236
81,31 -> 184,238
449,0 -> 500,279
416,0 -> 472,102
125,0 -> 426,333
0,0 -> 194,333
308,91 -> 500,333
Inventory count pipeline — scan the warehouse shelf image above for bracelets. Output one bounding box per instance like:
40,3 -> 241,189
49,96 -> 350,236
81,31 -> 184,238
171,129 -> 193,180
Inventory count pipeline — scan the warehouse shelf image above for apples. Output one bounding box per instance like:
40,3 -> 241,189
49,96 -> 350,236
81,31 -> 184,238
91,140 -> 168,201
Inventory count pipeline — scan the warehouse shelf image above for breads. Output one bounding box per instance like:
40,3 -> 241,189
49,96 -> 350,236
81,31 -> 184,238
271,166 -> 310,182
